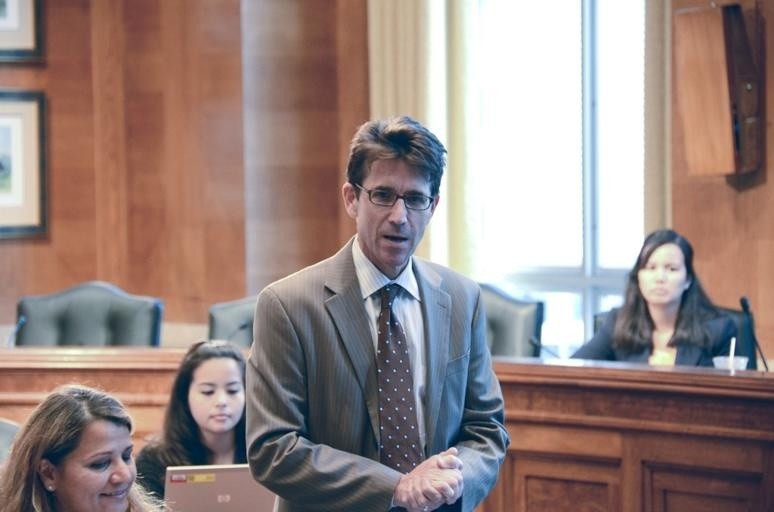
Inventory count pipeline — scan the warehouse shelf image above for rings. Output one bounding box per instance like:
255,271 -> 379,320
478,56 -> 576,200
423,504 -> 428,511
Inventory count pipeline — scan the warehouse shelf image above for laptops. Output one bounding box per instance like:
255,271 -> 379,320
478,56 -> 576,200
163,465 -> 278,512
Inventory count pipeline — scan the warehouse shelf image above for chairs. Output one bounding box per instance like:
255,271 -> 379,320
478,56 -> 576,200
472,280 -> 545,359
207,293 -> 272,346
592,307 -> 758,370
15,281 -> 171,343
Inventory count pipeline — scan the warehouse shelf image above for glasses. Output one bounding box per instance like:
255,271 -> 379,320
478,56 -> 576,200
351,183 -> 437,213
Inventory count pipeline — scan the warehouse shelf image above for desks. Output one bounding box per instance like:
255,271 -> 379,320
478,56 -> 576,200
2,346 -> 200,468
472,358 -> 773,511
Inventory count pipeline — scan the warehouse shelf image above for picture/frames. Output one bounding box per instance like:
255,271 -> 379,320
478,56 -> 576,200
0,88 -> 49,242
0,1 -> 48,64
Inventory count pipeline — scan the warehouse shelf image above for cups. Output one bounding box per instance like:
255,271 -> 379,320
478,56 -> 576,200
713,355 -> 748,371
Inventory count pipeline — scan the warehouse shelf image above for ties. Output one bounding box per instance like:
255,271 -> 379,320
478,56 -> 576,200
372,286 -> 427,474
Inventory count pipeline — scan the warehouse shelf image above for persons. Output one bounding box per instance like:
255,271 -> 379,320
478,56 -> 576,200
1,386 -> 171,512
247,118 -> 509,512
569,232 -> 739,373
134,341 -> 247,488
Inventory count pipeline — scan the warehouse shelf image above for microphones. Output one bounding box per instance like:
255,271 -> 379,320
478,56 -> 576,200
225,320 -> 250,340
528,335 -> 558,360
740,297 -> 769,374
